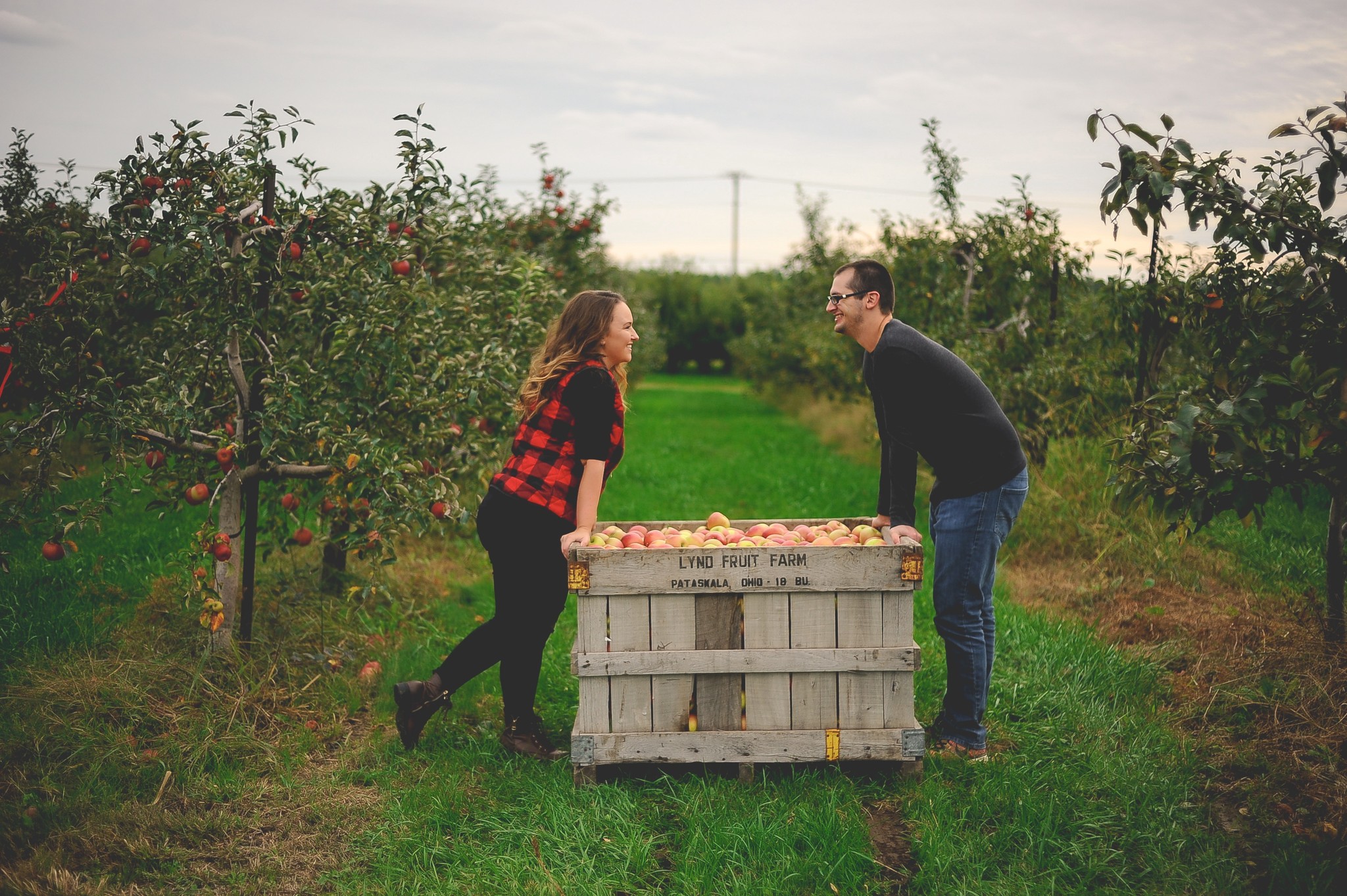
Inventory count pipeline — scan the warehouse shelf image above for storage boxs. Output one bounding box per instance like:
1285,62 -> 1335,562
564,516 -> 927,781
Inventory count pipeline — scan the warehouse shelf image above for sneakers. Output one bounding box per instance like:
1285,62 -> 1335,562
924,727 -> 940,748
928,738 -> 989,769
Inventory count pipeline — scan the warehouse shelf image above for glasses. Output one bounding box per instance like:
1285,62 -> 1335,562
827,290 -> 869,305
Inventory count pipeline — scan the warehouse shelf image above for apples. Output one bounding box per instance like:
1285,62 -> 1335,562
1023,209 -> 1033,220
1205,293 -> 1222,308
587,512 -> 888,549
1169,315 -> 1178,323
305,720 -> 318,731
0,176 -> 591,680
688,598 -> 746,732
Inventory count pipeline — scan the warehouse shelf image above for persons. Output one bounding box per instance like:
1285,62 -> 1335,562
394,290 -> 640,761
826,259 -> 1029,763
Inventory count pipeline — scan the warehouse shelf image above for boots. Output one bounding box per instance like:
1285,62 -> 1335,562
396,674 -> 450,751
502,707 -> 569,761
